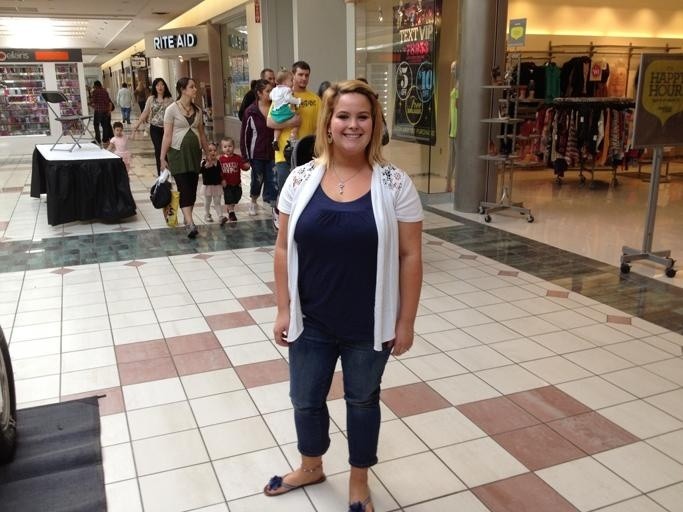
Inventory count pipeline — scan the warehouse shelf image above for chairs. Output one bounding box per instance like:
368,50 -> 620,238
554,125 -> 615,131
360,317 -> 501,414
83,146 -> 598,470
41,91 -> 103,152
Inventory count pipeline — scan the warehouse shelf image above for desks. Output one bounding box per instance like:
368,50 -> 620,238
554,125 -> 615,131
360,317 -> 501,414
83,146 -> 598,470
31,142 -> 137,226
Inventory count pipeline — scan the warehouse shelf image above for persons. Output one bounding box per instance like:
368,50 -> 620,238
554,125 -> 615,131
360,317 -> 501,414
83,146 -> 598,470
263,80 -> 425,512
445,61 -> 460,192
89,60 -> 389,238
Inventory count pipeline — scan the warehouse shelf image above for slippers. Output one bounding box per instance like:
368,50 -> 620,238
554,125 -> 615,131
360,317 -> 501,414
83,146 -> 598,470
349,496 -> 375,512
265,472 -> 325,498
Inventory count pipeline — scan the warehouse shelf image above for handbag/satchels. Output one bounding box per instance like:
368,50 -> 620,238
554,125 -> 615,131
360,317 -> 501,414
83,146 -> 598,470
144,125 -> 151,136
164,191 -> 179,226
150,181 -> 171,209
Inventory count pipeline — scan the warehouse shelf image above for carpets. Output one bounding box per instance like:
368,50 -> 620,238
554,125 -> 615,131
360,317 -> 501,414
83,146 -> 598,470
0,395 -> 109,512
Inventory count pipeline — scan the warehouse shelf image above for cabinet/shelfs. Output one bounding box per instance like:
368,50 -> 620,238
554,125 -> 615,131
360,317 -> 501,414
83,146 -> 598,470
503,40 -> 683,187
479,83 -> 533,222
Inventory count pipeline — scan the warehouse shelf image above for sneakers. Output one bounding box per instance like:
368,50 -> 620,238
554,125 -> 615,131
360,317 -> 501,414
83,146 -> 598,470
185,223 -> 198,238
228,209 -> 237,221
249,202 -> 257,215
204,214 -> 213,222
219,216 -> 227,225
272,141 -> 279,151
272,208 -> 280,229
